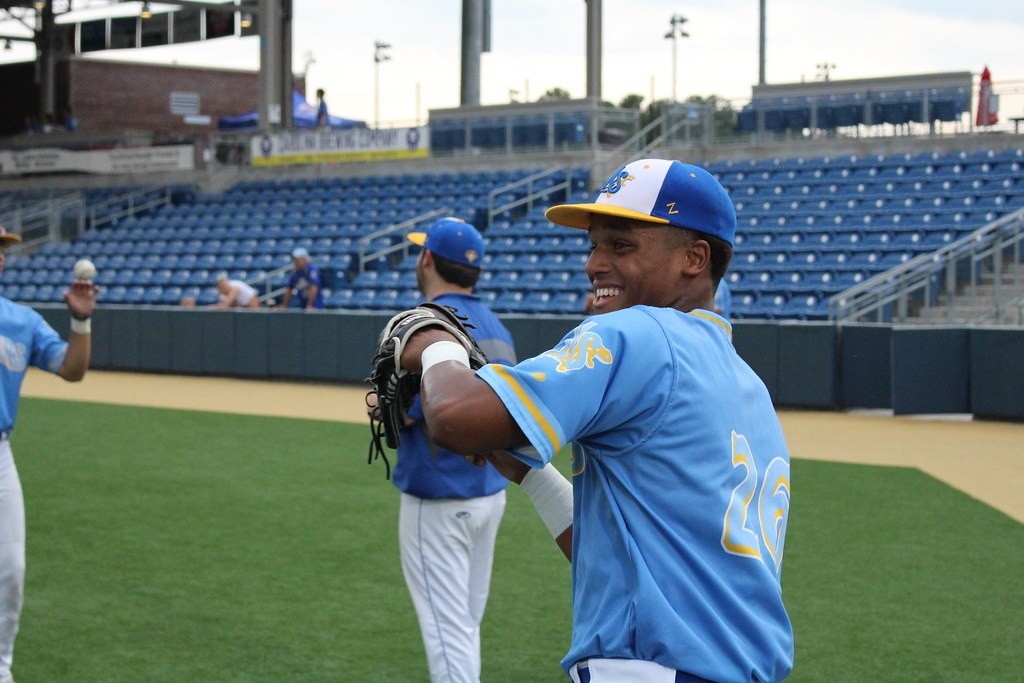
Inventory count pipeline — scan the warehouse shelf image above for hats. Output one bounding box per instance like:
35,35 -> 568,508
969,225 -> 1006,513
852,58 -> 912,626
407,217 -> 484,268
0,223 -> 21,248
544,158 -> 737,249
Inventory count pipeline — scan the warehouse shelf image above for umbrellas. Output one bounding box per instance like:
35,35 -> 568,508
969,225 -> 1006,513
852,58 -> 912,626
976,67 -> 998,131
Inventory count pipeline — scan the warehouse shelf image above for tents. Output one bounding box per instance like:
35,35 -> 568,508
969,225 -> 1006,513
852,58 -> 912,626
218,89 -> 366,130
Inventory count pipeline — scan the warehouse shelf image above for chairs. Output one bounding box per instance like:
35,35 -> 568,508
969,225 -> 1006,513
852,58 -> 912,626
0,88 -> 1024,322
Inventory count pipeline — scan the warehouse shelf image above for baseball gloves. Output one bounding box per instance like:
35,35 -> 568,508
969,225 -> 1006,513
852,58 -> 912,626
364,302 -> 490,448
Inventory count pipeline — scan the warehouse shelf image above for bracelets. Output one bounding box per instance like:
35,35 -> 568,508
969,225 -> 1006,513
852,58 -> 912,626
70,315 -> 91,333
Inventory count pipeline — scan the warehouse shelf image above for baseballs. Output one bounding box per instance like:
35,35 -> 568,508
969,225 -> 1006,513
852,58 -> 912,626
73,257 -> 97,281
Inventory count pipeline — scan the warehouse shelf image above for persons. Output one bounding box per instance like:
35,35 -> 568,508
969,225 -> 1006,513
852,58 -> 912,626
367,217 -> 518,683
209,272 -> 258,308
377,159 -> 795,683
275,247 -> 324,311
0,222 -> 99,683
314,89 -> 330,127
